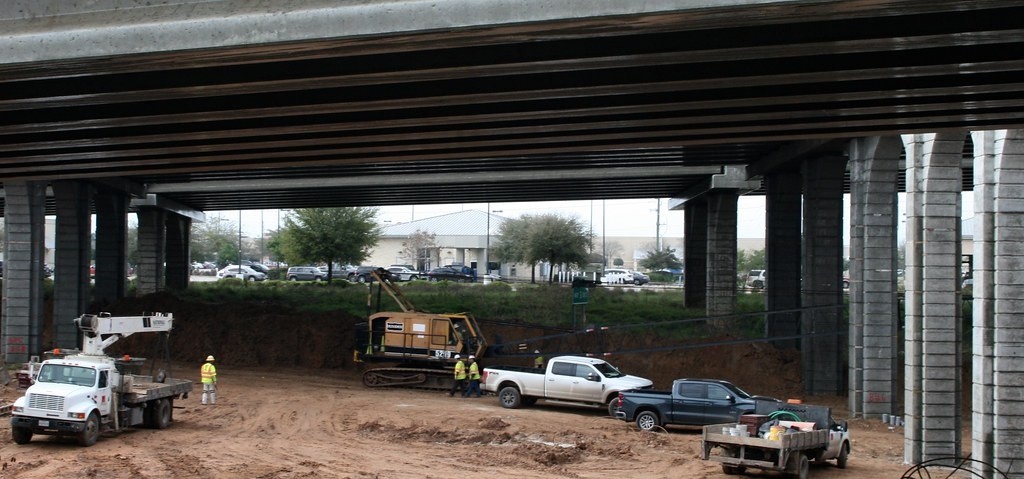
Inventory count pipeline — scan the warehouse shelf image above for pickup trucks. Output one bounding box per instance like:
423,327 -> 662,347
615,376 -> 783,433
478,354 -> 656,413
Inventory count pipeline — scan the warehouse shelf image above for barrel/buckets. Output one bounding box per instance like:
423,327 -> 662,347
722,424 -> 750,437
770,426 -> 785,441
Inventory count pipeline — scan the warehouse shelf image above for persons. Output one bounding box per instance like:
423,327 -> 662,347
467,355 -> 481,398
200,355 -> 217,405
446,354 -> 466,398
964,272 -> 970,281
452,323 -> 464,342
534,350 -> 544,368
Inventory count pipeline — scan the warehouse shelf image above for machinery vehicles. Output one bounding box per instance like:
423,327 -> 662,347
11,311 -> 195,447
351,266 -> 851,395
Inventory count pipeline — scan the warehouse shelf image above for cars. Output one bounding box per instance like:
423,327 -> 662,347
746,268 -> 767,290
318,263 -> 355,283
216,263 -> 268,282
603,267 -> 651,287
429,263 -> 478,284
246,260 -> 270,275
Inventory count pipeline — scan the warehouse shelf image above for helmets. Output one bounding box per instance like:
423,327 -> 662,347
534,350 -> 539,353
469,355 -> 475,359
454,324 -> 459,328
206,356 -> 215,361
454,354 -> 460,358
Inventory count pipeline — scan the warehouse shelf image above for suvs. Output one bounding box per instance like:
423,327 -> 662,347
385,264 -> 433,283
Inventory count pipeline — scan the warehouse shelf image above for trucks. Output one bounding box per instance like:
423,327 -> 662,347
701,400 -> 852,479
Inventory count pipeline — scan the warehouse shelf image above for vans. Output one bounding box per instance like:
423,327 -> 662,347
356,266 -> 398,284
286,266 -> 329,281
